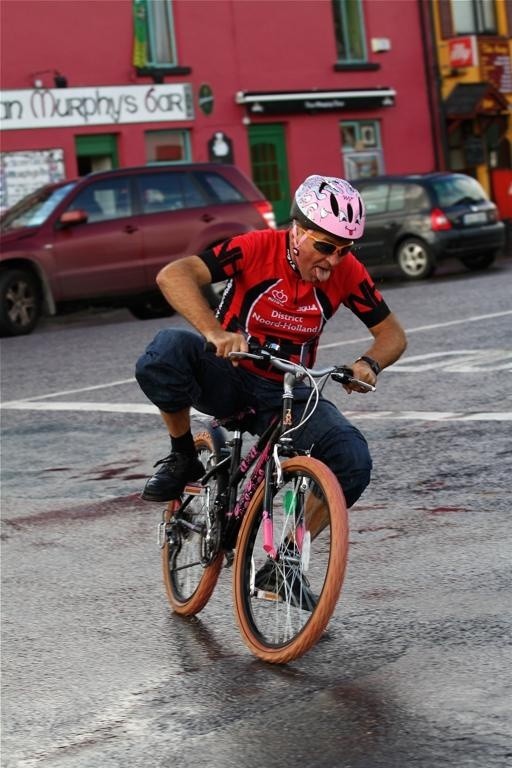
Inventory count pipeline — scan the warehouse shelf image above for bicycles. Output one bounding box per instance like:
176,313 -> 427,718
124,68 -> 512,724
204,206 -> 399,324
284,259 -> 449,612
157,341 -> 376,665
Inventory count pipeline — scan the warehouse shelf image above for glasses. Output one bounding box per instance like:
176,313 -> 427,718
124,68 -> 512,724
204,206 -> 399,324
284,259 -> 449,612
299,227 -> 355,258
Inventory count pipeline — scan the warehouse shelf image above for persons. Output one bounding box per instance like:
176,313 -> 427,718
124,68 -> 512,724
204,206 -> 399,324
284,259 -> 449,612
134,171 -> 411,616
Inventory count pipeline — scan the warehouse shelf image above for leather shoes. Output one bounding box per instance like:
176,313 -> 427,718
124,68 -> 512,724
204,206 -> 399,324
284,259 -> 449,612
139,451 -> 208,502
252,548 -> 318,613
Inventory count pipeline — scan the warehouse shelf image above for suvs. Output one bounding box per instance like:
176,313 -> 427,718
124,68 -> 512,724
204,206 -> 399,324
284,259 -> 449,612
0,162 -> 278,341
348,170 -> 506,280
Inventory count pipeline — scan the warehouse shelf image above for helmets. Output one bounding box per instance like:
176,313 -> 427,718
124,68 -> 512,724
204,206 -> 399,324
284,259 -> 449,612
290,172 -> 369,242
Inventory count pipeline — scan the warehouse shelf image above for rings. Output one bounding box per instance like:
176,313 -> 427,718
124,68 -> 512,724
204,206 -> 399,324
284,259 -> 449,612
359,385 -> 366,390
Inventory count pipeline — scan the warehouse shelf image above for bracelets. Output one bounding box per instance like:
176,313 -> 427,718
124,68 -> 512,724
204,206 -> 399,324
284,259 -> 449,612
357,356 -> 383,377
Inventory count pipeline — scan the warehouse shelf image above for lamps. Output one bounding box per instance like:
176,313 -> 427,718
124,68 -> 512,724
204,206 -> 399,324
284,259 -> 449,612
34,68 -> 66,87
